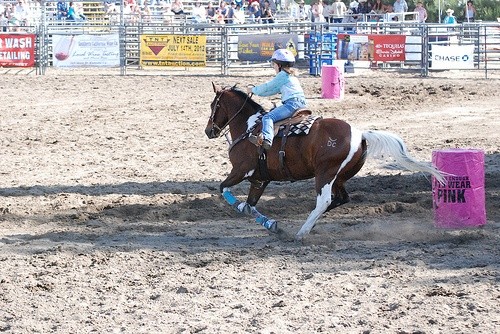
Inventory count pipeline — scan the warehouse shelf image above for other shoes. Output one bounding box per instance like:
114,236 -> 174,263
248,133 -> 272,150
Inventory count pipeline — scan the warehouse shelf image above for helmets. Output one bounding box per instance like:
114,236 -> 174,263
265,49 -> 295,68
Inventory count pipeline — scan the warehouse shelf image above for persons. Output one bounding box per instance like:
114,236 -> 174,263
247,49 -> 307,150
101,0 -> 245,30
0,0 -> 86,35
286,0 -> 428,34
247,0 -> 280,30
463,0 -> 477,22
442,8 -> 457,24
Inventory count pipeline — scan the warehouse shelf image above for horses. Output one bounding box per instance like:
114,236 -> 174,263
204,80 -> 456,241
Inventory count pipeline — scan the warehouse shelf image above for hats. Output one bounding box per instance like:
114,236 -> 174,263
237,3 -> 242,8
231,1 -> 237,5
250,1 -> 260,7
298,0 -> 305,4
445,8 -> 454,13
417,0 -> 423,4
